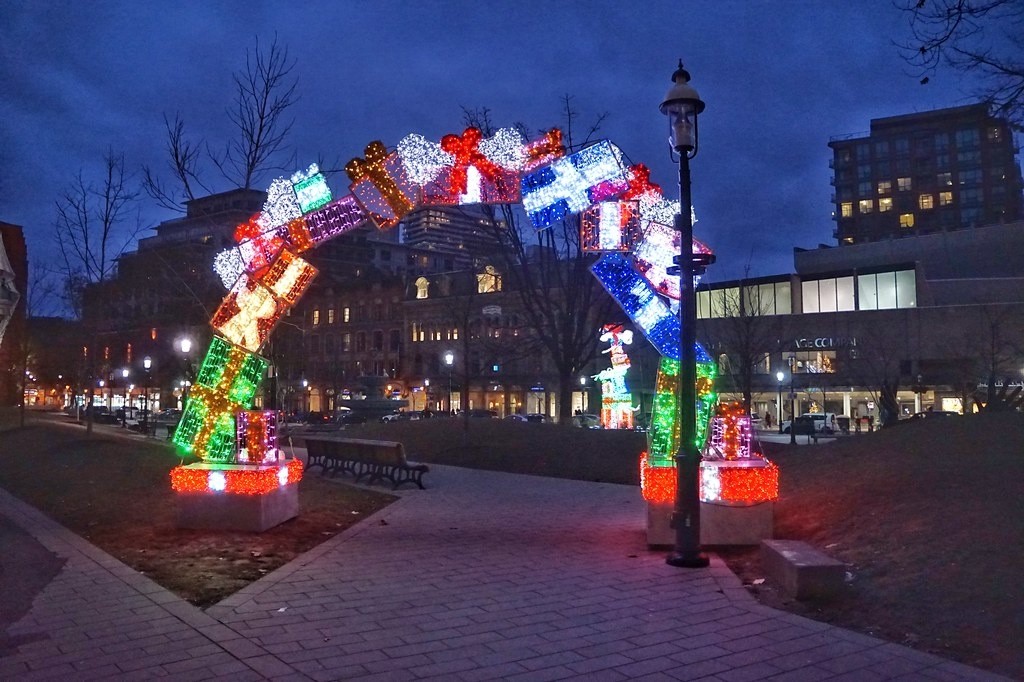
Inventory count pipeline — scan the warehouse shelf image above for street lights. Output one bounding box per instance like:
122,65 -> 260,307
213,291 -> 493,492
445,349 -> 454,415
787,354 -> 798,445
425,377 -> 430,410
141,355 -> 151,434
99,380 -> 104,406
776,372 -> 784,434
121,368 -> 129,429
580,374 -> 586,414
303,379 -> 308,411
658,56 -> 711,568
917,374 -> 923,417
180,339 -> 192,410
109,372 -> 114,415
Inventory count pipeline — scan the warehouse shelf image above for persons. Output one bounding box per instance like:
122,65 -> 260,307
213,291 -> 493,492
765,412 -> 771,428
421,410 -> 427,419
451,409 -> 455,415
576,409 -> 581,414
429,411 -> 434,419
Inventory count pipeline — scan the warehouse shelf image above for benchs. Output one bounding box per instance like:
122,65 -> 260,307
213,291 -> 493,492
303,436 -> 430,493
811,434 -> 852,443
166,423 -> 177,440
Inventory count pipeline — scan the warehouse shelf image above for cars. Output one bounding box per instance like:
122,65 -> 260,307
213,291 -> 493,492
572,414 -> 600,430
781,413 -> 837,433
63,406 -> 555,423
